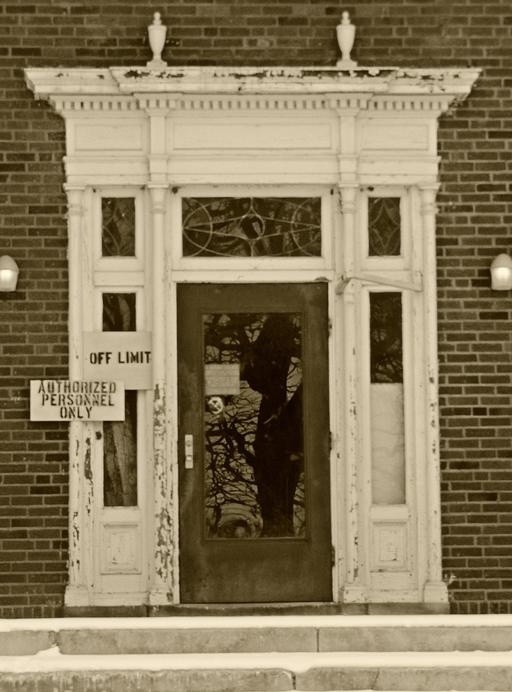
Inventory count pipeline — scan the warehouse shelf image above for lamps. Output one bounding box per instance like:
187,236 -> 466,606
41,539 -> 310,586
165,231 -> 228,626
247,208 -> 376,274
490,253 -> 512,291
0,254 -> 20,293
